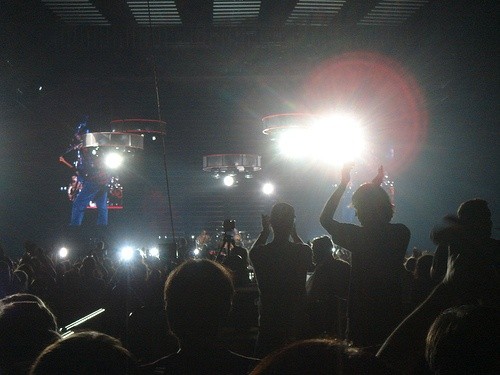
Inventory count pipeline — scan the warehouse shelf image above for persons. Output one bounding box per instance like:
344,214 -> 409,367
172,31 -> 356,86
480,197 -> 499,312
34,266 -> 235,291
0,198 -> 500,375
319,162 -> 411,354
70,128 -> 113,227
